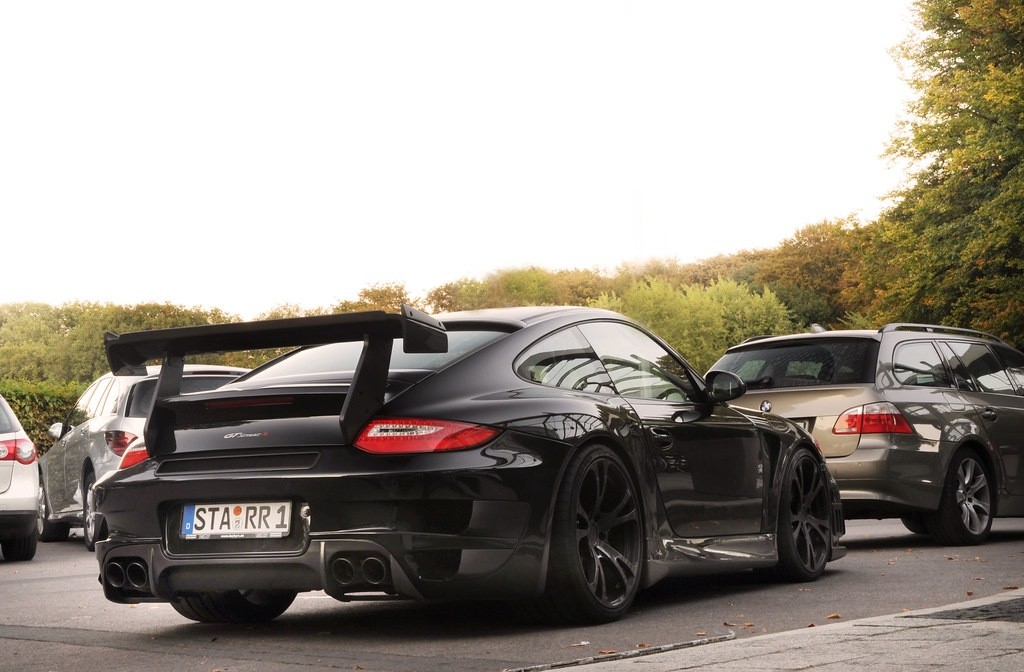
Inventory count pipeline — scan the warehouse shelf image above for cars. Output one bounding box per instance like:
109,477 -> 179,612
36,365 -> 252,554
0,395 -> 39,562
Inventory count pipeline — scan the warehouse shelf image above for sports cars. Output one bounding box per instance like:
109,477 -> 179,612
84,302 -> 847,625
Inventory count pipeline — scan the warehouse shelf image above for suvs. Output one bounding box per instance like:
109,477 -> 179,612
700,322 -> 1024,542
